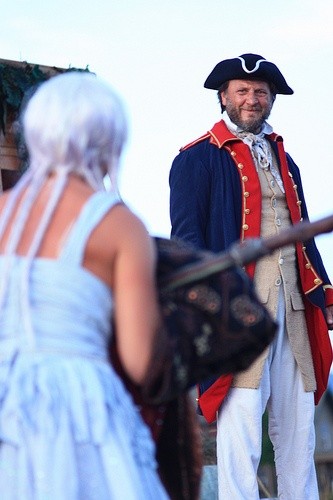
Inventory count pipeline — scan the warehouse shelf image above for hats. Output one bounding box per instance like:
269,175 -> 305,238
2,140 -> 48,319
204,53 -> 294,95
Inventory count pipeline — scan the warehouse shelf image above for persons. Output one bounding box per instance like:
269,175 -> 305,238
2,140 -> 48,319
0,71 -> 171,500
169,54 -> 333,500
